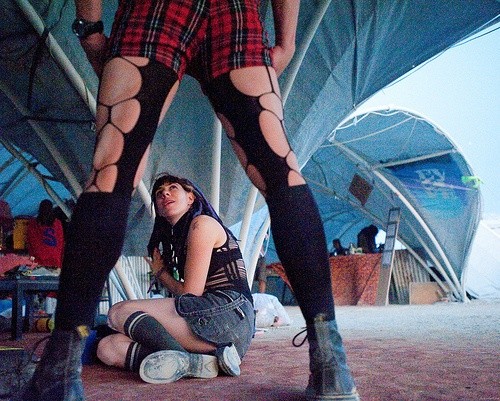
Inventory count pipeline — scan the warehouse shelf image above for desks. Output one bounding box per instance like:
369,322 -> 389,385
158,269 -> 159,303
0,280 -> 60,339
267,252 -> 381,305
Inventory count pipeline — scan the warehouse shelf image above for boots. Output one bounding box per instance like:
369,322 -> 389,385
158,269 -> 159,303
9,336 -> 85,401
292,319 -> 359,401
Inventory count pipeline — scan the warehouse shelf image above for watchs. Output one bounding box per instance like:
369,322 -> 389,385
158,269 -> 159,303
72,18 -> 104,37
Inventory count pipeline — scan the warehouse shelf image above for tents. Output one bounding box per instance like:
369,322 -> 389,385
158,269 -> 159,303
251,107 -> 482,304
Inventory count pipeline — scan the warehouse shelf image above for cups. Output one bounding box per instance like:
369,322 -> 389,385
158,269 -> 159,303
356,248 -> 362,254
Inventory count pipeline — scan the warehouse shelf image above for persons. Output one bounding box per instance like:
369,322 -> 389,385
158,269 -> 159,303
19,0 -> 360,401
24,199 -> 65,331
330,239 -> 346,256
96,175 -> 257,384
356,224 -> 379,253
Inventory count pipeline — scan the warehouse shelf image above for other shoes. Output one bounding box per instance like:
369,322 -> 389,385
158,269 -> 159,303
216,342 -> 241,376
139,349 -> 219,384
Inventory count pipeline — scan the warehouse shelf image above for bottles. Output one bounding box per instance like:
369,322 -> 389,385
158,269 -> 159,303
349,244 -> 355,254
